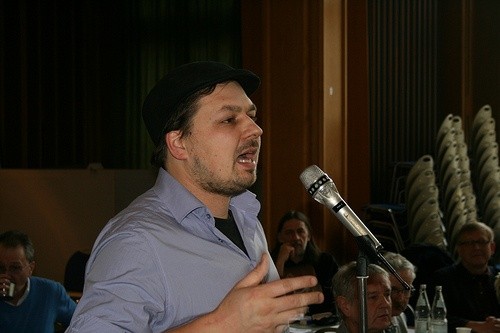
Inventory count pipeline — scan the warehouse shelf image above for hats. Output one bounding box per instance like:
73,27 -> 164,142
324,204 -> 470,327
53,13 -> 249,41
140,61 -> 260,166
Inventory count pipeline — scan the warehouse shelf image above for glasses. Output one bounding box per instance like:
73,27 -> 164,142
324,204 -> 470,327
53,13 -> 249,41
460,240 -> 490,246
391,286 -> 415,297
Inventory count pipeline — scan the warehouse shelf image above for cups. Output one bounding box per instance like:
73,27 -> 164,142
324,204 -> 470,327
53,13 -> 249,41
457,328 -> 472,333
0,280 -> 17,300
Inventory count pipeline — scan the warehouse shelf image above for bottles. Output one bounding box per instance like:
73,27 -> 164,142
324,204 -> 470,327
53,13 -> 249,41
430,286 -> 448,333
415,283 -> 431,333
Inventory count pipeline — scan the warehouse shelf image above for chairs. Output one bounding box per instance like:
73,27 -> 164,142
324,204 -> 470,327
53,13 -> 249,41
361,105 -> 500,259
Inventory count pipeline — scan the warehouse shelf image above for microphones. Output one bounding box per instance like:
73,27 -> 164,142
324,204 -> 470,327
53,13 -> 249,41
300,165 -> 410,290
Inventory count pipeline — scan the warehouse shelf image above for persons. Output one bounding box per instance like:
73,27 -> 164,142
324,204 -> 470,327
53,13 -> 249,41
0,231 -> 79,333
268,209 -> 337,324
367,251 -> 416,333
59,57 -> 326,333
425,222 -> 500,333
322,260 -> 392,333
